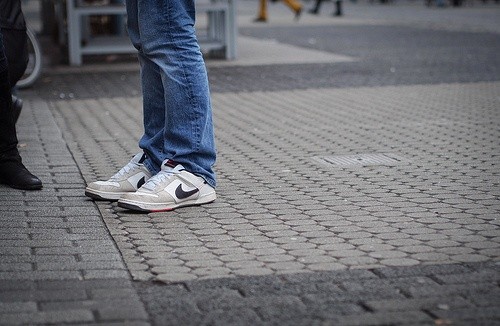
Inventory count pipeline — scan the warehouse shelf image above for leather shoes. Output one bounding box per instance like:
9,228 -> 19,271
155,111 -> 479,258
0,161 -> 43,190
12,94 -> 23,123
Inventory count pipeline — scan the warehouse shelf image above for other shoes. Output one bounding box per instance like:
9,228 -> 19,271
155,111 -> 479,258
295,7 -> 302,19
253,19 -> 267,23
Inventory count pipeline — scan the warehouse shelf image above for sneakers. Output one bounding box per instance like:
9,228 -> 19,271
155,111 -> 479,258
85,151 -> 152,200
118,159 -> 216,211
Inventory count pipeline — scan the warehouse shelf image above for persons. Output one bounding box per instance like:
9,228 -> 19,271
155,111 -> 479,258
84,0 -> 217,212
309,0 -> 343,17
0,0 -> 44,192
251,0 -> 304,24
